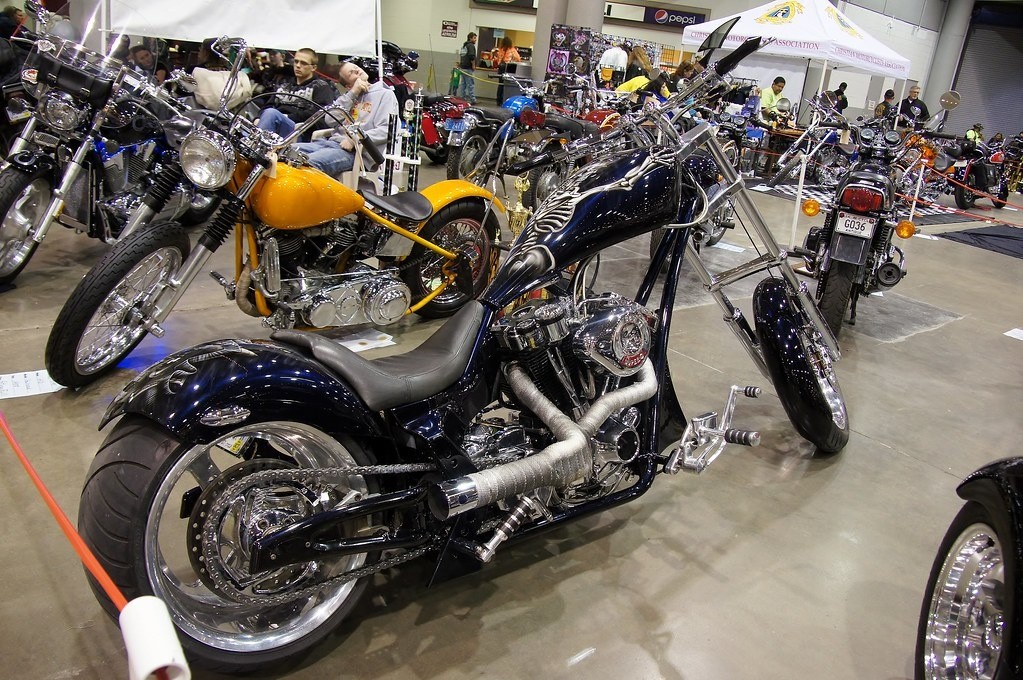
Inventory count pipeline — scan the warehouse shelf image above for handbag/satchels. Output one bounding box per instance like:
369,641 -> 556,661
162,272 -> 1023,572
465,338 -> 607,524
191,67 -> 252,111
498,62 -> 506,74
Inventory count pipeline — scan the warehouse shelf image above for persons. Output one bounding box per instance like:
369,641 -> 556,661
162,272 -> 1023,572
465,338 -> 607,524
0,0 -> 523,176
593,45 -> 1023,165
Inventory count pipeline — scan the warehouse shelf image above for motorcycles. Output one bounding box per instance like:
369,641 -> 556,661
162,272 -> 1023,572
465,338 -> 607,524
405,64 -> 1023,341
914,454 -> 1023,679
71,14 -> 852,680
0,1 -> 335,289
44,40 -> 508,395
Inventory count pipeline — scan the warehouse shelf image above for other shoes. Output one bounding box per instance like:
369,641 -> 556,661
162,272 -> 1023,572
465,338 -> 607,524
470,99 -> 481,105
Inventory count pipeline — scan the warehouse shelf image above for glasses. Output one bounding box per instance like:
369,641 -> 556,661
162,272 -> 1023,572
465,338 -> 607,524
292,60 -> 313,68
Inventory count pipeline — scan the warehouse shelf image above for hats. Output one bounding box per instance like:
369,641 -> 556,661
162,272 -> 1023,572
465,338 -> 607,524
973,122 -> 984,130
624,41 -> 633,52
265,49 -> 286,54
695,55 -> 705,62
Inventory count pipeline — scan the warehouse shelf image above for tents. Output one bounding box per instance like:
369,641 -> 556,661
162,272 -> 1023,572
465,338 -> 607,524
677,0 -> 912,155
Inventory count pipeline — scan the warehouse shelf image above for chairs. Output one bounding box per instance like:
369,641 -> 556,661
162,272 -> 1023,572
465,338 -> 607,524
255,118 -> 402,196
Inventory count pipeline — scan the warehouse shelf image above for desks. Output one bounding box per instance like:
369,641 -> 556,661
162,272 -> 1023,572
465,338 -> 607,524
751,129 -> 805,178
